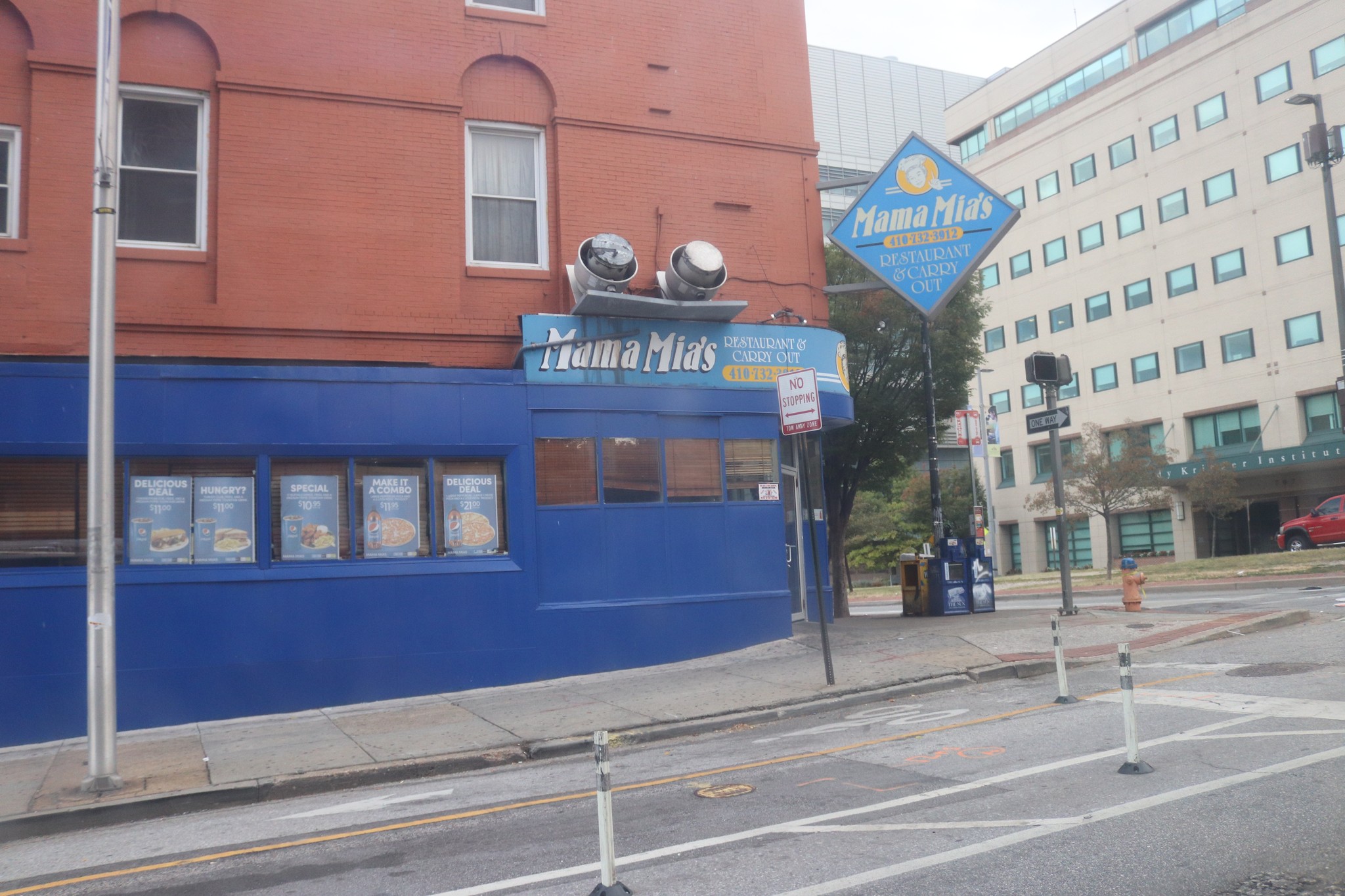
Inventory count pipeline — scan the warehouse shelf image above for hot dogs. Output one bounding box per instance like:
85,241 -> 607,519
150,529 -> 187,549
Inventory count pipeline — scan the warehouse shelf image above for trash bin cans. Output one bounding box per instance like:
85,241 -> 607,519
928,557 -> 970,615
966,557 -> 996,613
900,559 -> 930,616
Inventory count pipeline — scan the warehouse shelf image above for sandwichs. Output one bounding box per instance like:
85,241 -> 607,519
213,528 -> 248,545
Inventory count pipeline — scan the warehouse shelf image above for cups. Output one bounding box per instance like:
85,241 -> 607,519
195,518 -> 216,553
283,515 -> 303,547
131,518 -> 153,557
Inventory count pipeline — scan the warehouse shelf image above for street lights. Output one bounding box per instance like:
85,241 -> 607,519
1284,93 -> 1345,376
974,366 -> 1001,578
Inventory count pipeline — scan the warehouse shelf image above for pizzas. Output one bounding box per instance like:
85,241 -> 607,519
461,513 -> 489,525
381,518 -> 414,546
462,522 -> 495,546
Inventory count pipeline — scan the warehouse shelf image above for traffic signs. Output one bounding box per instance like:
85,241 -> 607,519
1025,404 -> 1073,435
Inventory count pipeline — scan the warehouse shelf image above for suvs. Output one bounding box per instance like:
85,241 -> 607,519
1277,493 -> 1345,551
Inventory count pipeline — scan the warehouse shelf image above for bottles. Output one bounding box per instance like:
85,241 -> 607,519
366,505 -> 382,550
448,504 -> 462,547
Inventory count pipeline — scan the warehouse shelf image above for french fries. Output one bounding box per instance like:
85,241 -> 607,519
217,539 -> 242,551
312,534 -> 335,548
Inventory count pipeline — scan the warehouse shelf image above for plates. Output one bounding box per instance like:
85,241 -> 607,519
463,522 -> 495,546
300,536 -> 335,549
149,537 -> 190,552
383,518 -> 416,547
214,539 -> 251,552
460,512 -> 490,524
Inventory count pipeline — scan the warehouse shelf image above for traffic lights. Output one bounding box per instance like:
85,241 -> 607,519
1024,351 -> 1074,386
